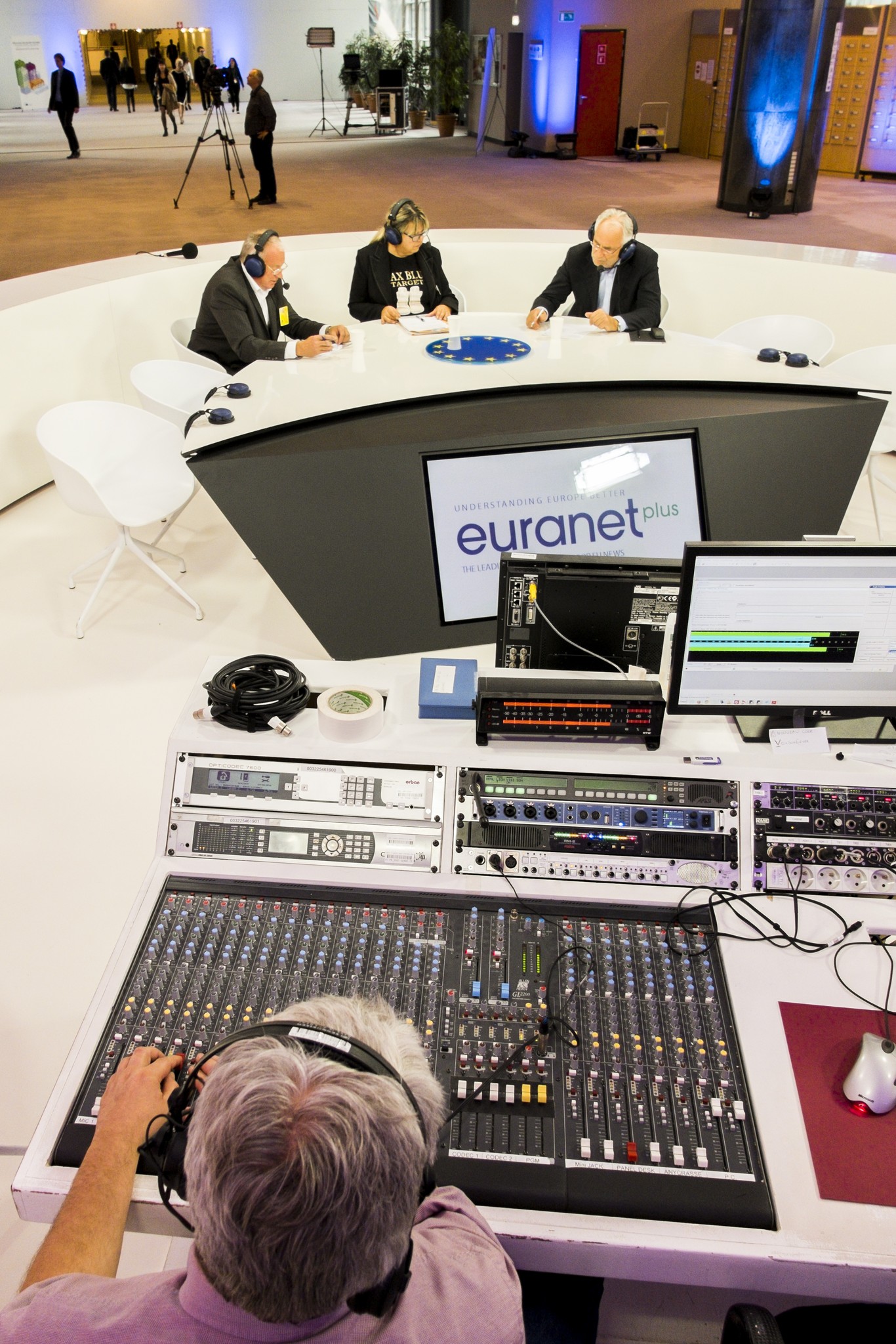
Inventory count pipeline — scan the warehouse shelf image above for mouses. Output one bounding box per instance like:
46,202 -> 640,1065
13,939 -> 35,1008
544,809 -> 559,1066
842,1032 -> 896,1116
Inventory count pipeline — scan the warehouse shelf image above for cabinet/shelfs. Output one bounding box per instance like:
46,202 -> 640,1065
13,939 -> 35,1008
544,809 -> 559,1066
678,4 -> 896,185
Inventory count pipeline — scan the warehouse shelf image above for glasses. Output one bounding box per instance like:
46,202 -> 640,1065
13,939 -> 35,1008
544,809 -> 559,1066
199,50 -> 205,53
590,239 -> 624,256
403,229 -> 432,242
265,261 -> 288,276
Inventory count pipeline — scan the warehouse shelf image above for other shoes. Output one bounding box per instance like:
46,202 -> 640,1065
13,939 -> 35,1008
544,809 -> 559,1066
110,106 -> 113,111
174,125 -> 177,134
128,108 -> 131,113
132,107 -> 136,112
204,108 -> 208,111
258,197 -> 277,205
67,148 -> 80,158
185,107 -> 188,111
250,194 -> 260,202
162,130 -> 168,136
188,105 -> 192,110
155,107 -> 159,111
233,104 -> 235,112
237,110 -> 241,114
114,109 -> 119,112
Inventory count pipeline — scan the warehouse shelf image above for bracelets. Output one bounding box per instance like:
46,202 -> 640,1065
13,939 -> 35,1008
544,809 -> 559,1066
325,326 -> 331,335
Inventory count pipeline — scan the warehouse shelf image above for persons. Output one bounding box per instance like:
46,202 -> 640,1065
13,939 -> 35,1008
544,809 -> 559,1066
180,51 -> 194,111
154,61 -> 179,137
48,53 -> 80,159
99,50 -> 120,112
0,994 -> 527,1344
155,41 -> 166,66
119,57 -> 137,113
227,58 -> 244,114
188,229 -> 350,376
347,198 -> 459,325
109,47 -> 121,69
171,58 -> 187,124
526,208 -> 661,332
145,47 -> 163,112
244,69 -> 277,205
166,39 -> 178,70
194,46 -> 211,116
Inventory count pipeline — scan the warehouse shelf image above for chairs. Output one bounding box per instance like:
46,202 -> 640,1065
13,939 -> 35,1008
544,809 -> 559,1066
33,314 -> 236,643
725,313 -> 834,365
561,292 -> 669,328
822,348 -> 896,544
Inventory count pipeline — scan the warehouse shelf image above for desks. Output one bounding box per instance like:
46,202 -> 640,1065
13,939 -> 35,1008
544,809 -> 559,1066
180,310 -> 889,661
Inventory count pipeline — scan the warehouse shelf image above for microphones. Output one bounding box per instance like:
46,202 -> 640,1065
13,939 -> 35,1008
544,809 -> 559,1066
597,258 -> 629,273
159,242 -> 198,259
282,278 -> 290,290
425,235 -> 431,248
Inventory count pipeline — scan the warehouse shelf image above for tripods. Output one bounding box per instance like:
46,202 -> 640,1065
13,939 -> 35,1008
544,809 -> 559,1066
343,72 -> 376,135
174,88 -> 253,209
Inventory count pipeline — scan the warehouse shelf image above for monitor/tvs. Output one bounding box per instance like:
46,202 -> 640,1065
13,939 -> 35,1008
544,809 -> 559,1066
496,552 -> 683,674
423,428 -> 711,627
666,542 -> 896,744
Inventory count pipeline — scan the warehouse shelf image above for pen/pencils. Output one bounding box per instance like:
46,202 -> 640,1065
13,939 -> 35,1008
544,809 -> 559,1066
416,315 -> 425,322
322,337 -> 327,341
530,308 -> 544,327
638,330 -> 641,339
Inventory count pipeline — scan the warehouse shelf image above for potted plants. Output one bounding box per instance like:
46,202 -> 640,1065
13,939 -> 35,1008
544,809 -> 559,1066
335,17 -> 477,138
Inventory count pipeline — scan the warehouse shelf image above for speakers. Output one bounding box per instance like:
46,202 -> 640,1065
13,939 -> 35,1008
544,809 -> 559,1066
379,70 -> 407,87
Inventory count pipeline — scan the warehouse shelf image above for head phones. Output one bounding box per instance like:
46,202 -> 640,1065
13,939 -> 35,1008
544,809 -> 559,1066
204,383 -> 251,404
385,198 -> 414,246
184,408 -> 234,439
757,349 -> 792,362
243,230 -> 279,278
785,353 -> 820,367
137,1019 -> 435,1319
588,208 -> 638,261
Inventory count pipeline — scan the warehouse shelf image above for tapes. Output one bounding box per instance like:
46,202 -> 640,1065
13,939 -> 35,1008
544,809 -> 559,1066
316,685 -> 386,741
